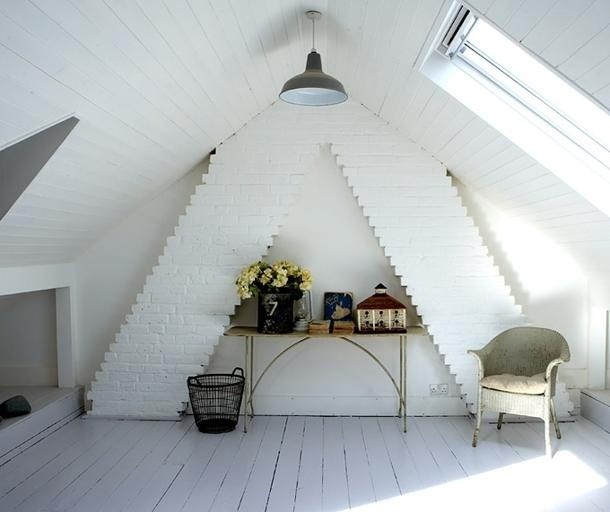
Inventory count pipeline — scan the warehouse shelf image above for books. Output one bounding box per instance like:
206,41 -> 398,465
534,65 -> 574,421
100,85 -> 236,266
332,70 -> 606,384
333,320 -> 356,329
309,318 -> 333,330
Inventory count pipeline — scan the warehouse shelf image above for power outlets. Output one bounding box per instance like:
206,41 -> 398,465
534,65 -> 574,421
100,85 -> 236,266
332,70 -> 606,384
439,384 -> 448,393
429,384 -> 438,395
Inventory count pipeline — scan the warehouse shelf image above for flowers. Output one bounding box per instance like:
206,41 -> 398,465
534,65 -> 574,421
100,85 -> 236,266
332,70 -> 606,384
234,261 -> 311,300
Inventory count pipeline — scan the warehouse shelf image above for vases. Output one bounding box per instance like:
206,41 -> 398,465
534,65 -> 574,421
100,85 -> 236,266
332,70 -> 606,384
258,290 -> 296,332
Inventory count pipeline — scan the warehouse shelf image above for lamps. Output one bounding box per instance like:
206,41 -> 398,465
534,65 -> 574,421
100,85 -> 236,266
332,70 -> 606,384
279,10 -> 348,107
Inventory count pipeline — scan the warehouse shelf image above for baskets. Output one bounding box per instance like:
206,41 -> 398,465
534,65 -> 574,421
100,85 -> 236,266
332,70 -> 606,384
186,367 -> 245,434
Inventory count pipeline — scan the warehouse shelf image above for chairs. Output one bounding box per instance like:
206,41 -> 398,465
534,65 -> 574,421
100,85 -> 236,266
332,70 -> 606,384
466,326 -> 570,458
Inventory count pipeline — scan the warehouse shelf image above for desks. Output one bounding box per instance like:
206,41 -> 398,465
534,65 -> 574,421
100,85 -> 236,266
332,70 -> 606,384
223,325 -> 429,432
